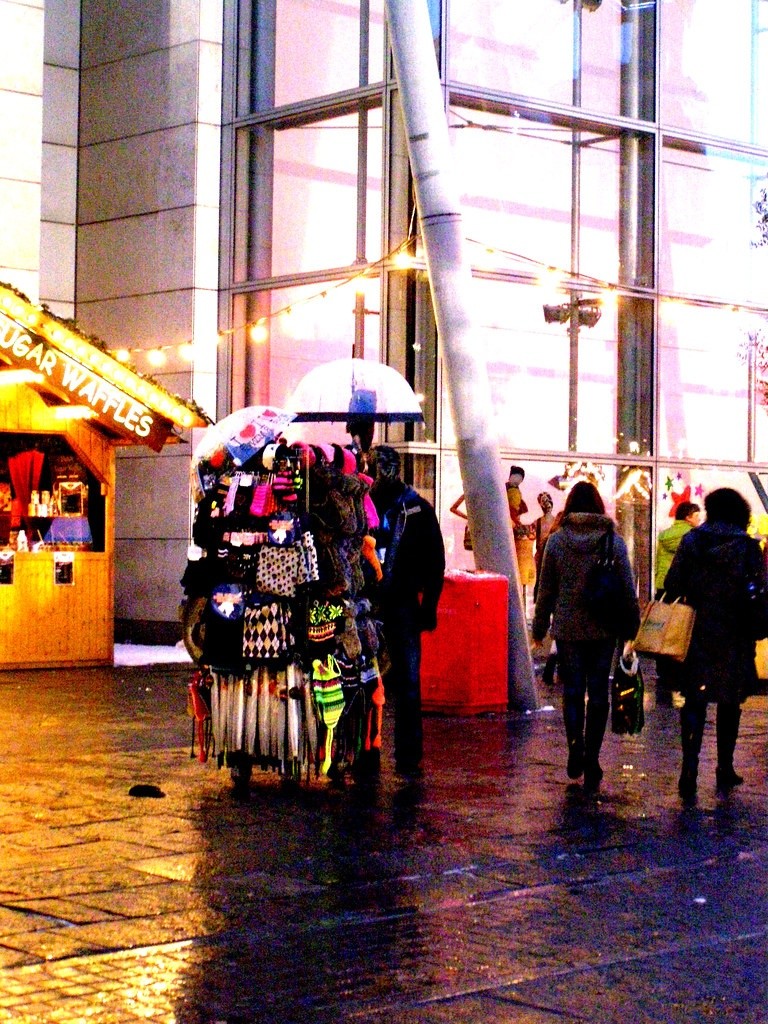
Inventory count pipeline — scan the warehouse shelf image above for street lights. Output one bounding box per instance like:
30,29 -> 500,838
543,296 -> 602,505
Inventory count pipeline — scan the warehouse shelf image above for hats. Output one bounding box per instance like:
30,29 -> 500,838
373,446 -> 401,467
291,441 -> 386,777
187,669 -> 217,762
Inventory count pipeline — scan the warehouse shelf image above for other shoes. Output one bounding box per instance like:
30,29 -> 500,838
345,748 -> 381,780
395,760 -> 421,778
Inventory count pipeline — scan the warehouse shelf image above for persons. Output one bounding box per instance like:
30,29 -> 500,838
352,446 -> 445,783
449,495 -> 468,520
504,467 -> 535,617
2,436 -> 57,543
531,481 -> 641,782
531,491 -> 557,605
651,502 -> 701,705
662,488 -> 768,796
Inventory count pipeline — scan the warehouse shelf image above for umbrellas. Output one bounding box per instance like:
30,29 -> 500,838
189,404 -> 297,501
206,652 -> 322,783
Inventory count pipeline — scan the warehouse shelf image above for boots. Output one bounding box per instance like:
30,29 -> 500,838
678,708 -> 706,803
563,700 -> 584,778
584,701 -> 610,789
715,706 -> 743,791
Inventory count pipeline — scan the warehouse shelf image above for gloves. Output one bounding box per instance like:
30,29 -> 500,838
420,597 -> 437,633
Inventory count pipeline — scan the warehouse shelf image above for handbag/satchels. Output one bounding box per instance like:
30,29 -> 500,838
182,447 -> 321,680
741,539 -> 768,639
633,591 -> 698,665
576,530 -> 641,642
610,638 -> 645,735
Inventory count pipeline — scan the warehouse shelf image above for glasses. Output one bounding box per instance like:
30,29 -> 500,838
375,465 -> 396,477
